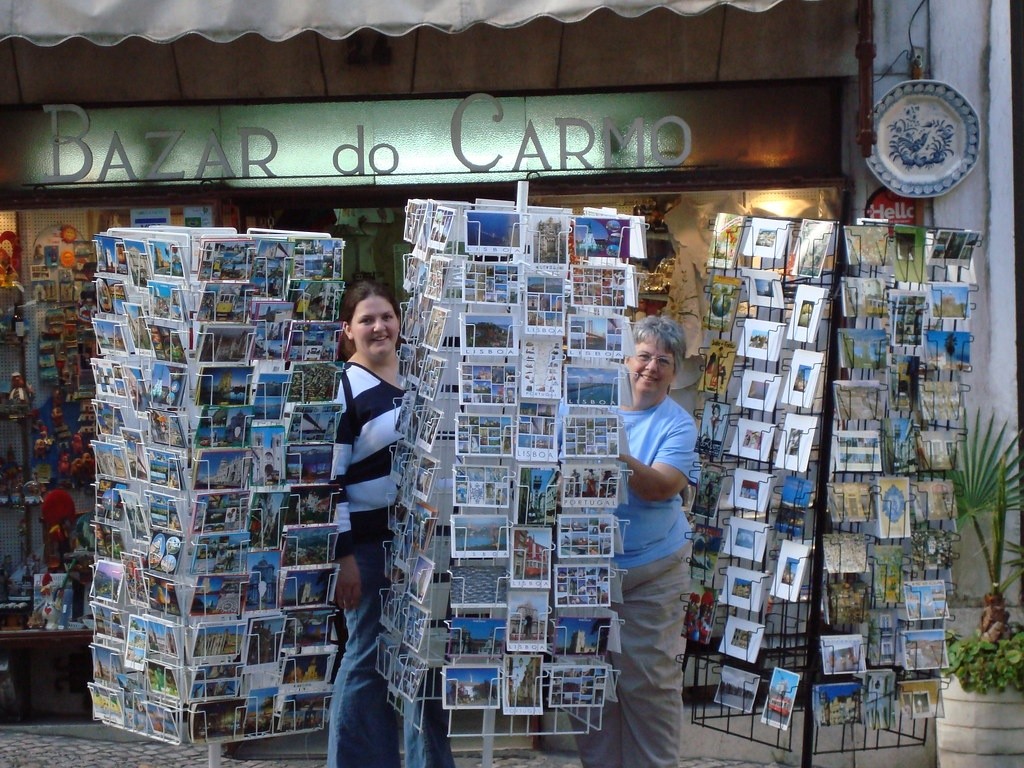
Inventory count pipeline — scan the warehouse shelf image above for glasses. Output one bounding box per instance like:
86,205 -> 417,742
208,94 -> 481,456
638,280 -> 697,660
634,350 -> 675,370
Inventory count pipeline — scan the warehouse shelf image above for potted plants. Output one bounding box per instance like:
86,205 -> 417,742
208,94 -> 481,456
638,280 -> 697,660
917,407 -> 1024,757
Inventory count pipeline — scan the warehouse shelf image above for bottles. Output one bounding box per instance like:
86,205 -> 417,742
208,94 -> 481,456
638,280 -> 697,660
12,303 -> 24,343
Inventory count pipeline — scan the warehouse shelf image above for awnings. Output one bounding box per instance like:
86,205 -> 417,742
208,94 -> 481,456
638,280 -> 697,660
0,0 -> 794,49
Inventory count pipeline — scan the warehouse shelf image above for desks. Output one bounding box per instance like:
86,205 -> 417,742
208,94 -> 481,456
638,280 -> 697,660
0,628 -> 99,726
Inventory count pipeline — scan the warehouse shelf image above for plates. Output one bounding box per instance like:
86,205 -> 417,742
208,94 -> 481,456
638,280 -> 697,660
865,79 -> 981,198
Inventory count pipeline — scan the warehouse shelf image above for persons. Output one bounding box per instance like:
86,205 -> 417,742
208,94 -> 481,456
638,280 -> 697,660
325,281 -> 461,768
511,658 -> 538,709
504,388 -> 515,403
525,615 -> 534,639
567,313 -> 703,768
197,536 -> 237,573
455,468 -> 507,506
567,469 -> 616,498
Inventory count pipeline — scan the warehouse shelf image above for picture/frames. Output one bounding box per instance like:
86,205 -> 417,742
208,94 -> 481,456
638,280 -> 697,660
728,468 -> 776,513
741,268 -> 785,309
720,565 -> 768,613
729,417 -> 777,463
744,218 -> 791,260
770,539 -> 812,603
775,413 -> 819,473
781,348 -> 825,408
736,318 -> 787,362
724,515 -> 769,562
787,283 -> 829,343
736,370 -> 783,413
718,616 -> 765,664
